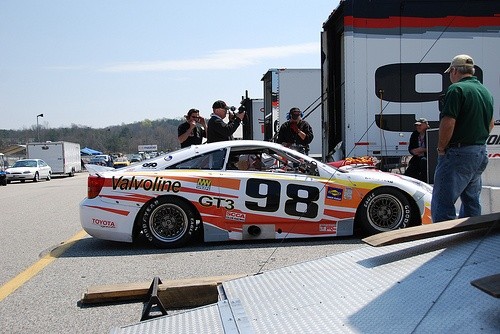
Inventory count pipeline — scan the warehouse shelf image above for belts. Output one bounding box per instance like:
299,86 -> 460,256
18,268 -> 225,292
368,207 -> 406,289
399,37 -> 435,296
449,143 -> 484,148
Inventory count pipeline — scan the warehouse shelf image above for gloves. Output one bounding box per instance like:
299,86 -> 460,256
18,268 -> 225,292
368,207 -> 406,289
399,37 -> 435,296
291,121 -> 301,134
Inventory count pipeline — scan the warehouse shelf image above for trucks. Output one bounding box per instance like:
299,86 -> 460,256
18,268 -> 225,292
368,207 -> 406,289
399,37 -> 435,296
25,140 -> 81,177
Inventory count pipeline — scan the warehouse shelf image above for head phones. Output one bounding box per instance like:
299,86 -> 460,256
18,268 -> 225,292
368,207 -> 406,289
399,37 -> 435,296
286,111 -> 304,120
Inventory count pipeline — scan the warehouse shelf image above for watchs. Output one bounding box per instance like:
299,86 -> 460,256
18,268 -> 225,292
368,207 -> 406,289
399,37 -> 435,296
437,149 -> 444,153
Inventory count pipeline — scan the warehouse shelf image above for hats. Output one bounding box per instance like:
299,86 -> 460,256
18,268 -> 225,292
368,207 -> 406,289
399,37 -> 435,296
290,108 -> 301,112
415,118 -> 428,125
212,100 -> 231,110
445,54 -> 474,73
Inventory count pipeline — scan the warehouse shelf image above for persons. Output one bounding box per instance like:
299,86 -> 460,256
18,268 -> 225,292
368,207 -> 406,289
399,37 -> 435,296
278,108 -> 313,156
207,100 -> 246,143
178,109 -> 208,149
404,118 -> 431,182
430,54 -> 493,223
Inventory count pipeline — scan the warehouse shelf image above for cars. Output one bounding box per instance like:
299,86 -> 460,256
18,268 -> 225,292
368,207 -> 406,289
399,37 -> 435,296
5,158 -> 53,182
78,138 -> 434,251
0,152 -> 9,186
80,150 -> 163,169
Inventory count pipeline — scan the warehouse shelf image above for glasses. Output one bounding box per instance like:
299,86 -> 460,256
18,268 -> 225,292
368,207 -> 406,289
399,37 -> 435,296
190,116 -> 199,118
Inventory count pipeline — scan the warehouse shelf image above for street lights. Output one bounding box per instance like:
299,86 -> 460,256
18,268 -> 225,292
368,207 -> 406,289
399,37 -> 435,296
36,113 -> 43,142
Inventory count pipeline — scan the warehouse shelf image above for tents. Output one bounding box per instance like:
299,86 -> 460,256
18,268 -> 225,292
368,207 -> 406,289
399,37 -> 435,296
80,147 -> 103,156
